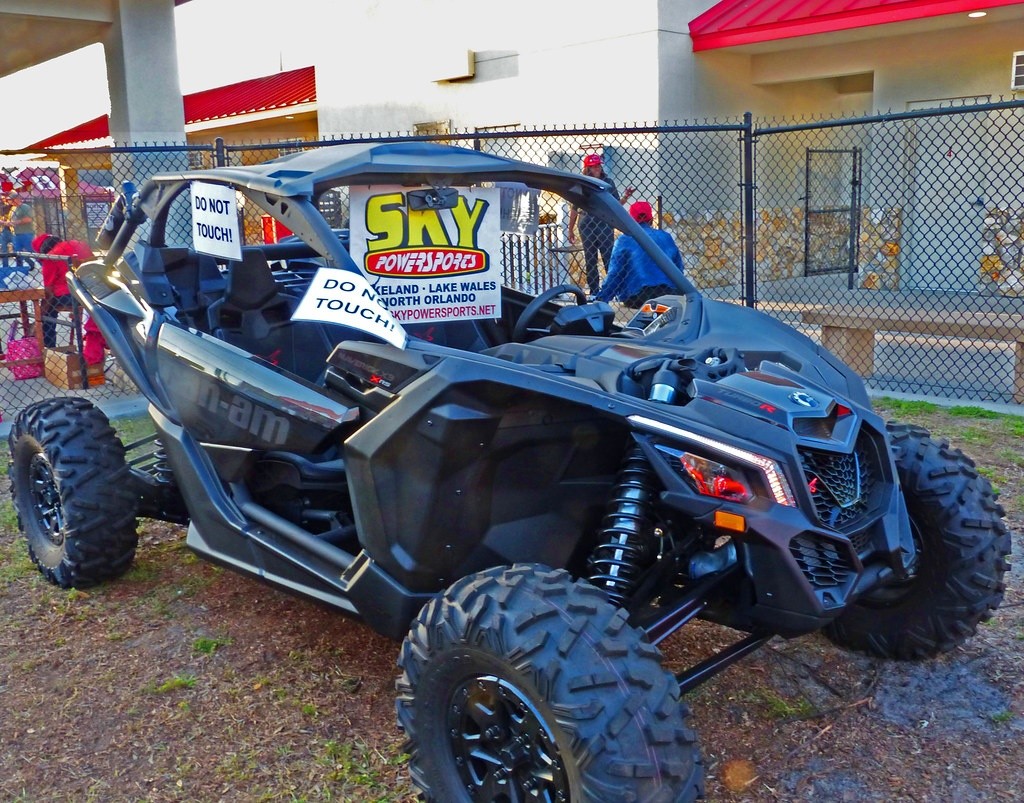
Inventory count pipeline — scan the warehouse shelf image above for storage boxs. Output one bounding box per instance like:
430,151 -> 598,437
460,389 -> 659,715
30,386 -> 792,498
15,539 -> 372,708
40,345 -> 106,390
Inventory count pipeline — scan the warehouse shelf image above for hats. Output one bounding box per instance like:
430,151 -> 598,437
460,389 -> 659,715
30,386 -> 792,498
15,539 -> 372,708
582,154 -> 605,168
629,201 -> 654,224
32,232 -> 52,253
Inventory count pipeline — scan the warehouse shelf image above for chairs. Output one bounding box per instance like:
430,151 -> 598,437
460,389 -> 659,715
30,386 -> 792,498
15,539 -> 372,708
205,246 -> 333,388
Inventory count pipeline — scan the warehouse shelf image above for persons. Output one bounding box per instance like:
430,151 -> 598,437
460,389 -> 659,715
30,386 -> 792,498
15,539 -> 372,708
0,193 -> 36,271
590,201 -> 685,310
568,155 -> 637,300
31,234 -> 99,349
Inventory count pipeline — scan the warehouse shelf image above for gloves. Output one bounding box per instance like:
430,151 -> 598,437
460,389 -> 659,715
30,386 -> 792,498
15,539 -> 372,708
624,185 -> 638,200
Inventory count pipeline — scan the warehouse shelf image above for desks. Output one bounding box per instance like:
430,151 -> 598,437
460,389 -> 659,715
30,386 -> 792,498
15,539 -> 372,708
0,289 -> 46,368
548,245 -> 602,303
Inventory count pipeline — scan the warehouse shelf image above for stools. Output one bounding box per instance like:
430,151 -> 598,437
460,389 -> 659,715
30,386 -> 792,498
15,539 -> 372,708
54,306 -> 85,345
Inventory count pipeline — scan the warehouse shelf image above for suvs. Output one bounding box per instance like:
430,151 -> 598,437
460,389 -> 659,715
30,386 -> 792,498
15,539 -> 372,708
5,132 -> 1016,803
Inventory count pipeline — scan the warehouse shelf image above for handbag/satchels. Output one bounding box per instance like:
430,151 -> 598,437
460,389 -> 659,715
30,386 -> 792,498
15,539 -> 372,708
5,320 -> 46,381
